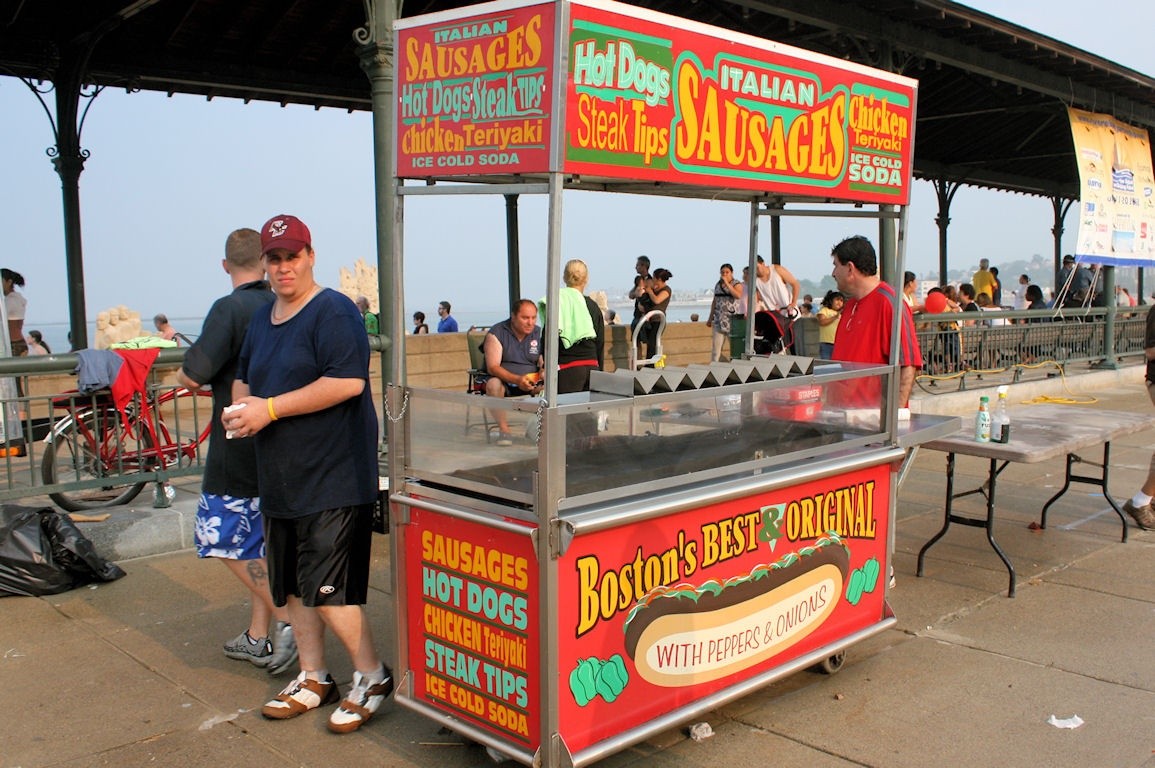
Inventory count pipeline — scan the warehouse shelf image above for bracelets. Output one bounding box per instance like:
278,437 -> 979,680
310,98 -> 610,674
268,398 -> 277,421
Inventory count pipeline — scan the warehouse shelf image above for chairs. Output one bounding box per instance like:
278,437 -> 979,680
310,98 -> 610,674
465,327 -> 532,444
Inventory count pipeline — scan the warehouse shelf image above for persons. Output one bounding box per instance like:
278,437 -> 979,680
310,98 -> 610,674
474,299 -> 544,445
412,311 -> 429,335
690,235 -> 1155,588
175,229 -> 300,675
0,268 -> 52,410
437,300 -> 458,332
355,295 -> 378,335
153,313 -> 181,347
628,255 -> 673,372
221,213 -> 393,732
537,259 -> 604,394
604,310 -> 616,325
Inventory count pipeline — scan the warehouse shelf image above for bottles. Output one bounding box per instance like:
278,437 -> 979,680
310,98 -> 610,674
976,396 -> 992,443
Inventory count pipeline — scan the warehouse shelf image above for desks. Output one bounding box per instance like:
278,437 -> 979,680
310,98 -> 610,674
917,403 -> 1155,599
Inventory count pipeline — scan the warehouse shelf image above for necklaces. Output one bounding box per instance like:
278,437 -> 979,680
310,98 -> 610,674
274,287 -> 312,320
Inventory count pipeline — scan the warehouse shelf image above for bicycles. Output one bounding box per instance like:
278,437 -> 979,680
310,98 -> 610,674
41,331 -> 214,515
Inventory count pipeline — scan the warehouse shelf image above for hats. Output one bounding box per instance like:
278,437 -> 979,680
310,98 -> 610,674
259,215 -> 311,260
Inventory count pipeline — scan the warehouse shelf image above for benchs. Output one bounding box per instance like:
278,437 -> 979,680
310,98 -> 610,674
911,305 -> 1154,375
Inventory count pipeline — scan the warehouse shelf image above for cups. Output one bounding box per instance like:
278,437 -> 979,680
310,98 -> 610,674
224,403 -> 249,438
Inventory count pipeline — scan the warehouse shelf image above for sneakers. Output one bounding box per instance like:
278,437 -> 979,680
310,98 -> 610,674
222,628 -> 273,667
261,670 -> 339,719
328,662 -> 394,733
1123,499 -> 1155,531
267,621 -> 298,675
496,432 -> 512,446
